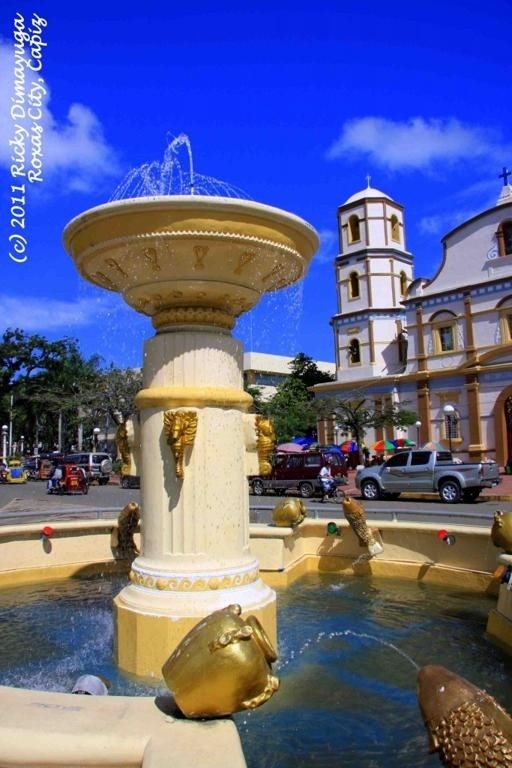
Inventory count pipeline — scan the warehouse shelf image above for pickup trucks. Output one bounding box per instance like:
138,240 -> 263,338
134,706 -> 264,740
355,448 -> 503,503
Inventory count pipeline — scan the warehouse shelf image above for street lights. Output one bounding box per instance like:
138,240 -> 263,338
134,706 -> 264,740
93,427 -> 101,451
415,420 -> 422,448
444,404 -> 455,454
334,425 -> 340,445
2,424 -> 25,460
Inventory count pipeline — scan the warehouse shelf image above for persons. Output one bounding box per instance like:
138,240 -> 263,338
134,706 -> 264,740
318,466 -> 338,502
47,464 -> 64,490
374,455 -> 386,465
364,445 -> 371,463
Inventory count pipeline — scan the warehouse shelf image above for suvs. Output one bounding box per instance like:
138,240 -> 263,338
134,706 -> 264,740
0,451 -> 113,497
249,448 -> 349,497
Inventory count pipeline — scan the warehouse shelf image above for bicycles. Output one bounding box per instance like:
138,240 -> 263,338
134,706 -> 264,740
310,475 -> 346,503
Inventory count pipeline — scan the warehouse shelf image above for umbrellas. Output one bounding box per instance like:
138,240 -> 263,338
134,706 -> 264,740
275,431 -> 358,465
397,439 -> 417,451
372,437 -> 395,457
